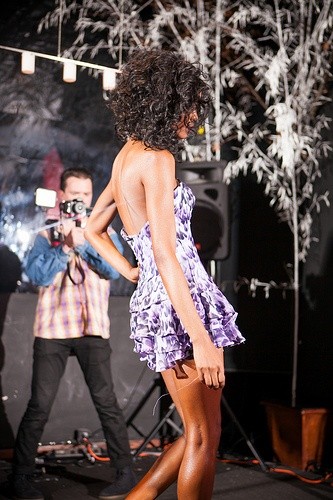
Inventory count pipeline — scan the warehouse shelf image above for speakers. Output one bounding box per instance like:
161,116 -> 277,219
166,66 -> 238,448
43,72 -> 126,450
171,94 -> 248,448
175,160 -> 232,260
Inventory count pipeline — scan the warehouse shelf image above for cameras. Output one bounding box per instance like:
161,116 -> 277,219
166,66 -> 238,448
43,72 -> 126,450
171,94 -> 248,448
60,198 -> 86,214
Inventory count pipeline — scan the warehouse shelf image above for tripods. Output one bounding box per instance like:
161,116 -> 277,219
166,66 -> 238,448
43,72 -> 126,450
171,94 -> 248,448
131,390 -> 268,475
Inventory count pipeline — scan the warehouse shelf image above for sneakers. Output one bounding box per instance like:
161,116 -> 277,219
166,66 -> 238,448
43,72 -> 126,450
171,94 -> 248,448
5,481 -> 44,500
99,468 -> 138,500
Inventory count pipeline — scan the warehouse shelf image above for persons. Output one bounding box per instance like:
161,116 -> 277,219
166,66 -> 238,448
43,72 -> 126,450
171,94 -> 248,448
4,167 -> 140,500
83,48 -> 247,500
